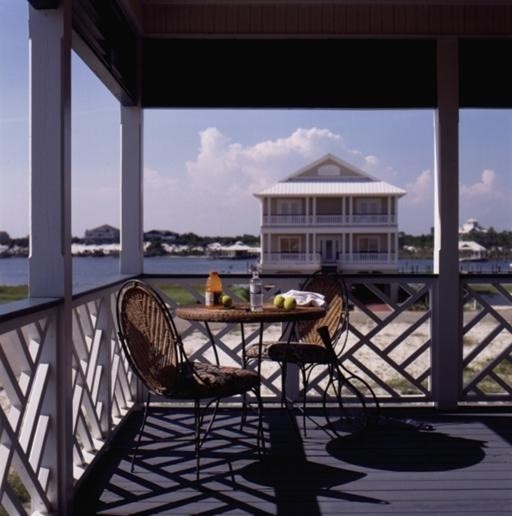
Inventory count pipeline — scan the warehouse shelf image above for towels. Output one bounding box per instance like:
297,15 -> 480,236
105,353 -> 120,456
275,289 -> 326,308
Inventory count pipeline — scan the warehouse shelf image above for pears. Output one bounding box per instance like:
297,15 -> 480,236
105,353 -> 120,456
284,298 -> 296,310
274,294 -> 283,308
222,295 -> 231,307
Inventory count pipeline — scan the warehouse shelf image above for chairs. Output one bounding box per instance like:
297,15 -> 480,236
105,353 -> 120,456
245,268 -> 350,439
117,279 -> 265,492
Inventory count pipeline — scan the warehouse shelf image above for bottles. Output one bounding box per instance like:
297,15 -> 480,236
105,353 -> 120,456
203,271 -> 223,308
249,272 -> 263,312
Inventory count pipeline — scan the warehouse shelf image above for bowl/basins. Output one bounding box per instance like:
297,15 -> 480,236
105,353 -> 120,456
225,284 -> 276,305
